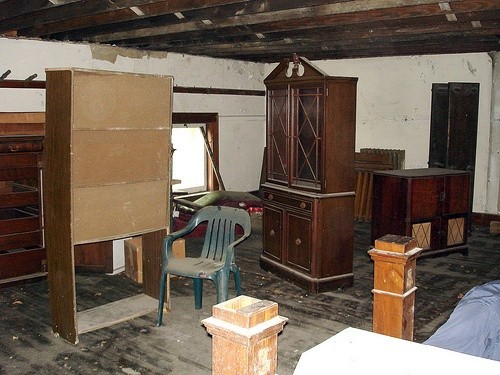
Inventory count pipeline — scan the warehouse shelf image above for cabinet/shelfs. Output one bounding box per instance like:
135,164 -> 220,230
2,112 -> 49,285
368,167 -> 472,260
43,66 -> 174,343
260,51 -> 358,293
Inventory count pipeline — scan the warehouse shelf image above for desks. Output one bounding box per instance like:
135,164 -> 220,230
292,326 -> 500,374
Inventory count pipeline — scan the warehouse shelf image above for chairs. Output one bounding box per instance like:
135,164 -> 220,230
155,206 -> 254,329
